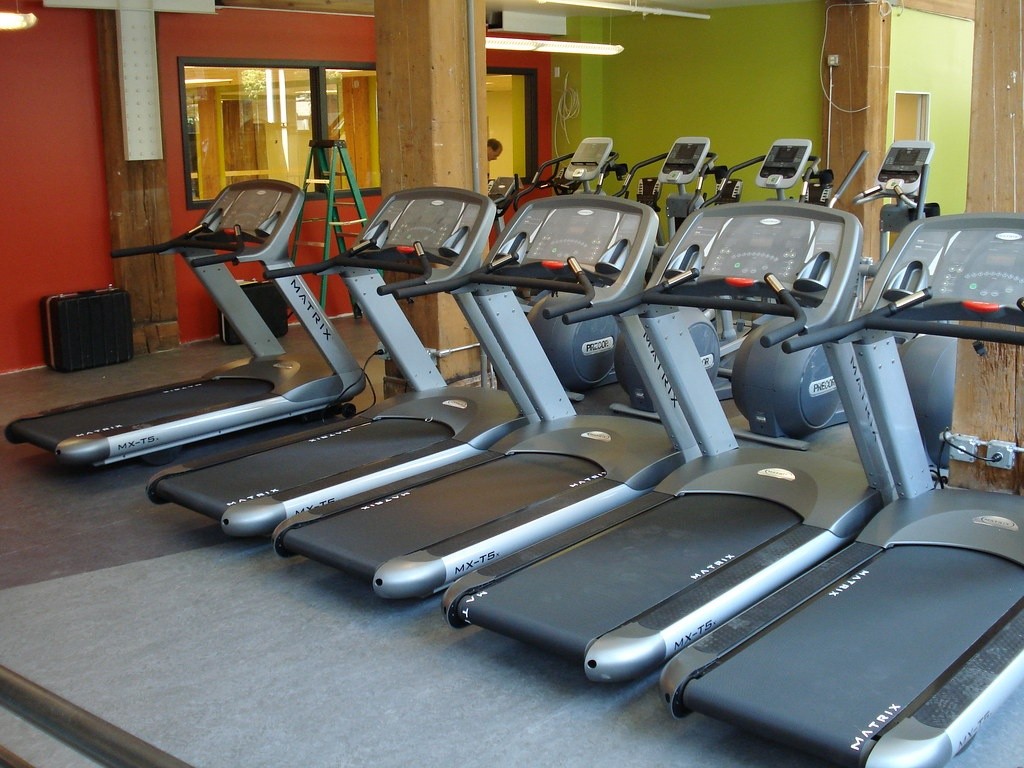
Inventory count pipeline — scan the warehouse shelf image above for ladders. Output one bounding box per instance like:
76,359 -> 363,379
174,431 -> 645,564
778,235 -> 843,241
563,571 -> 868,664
291,139 -> 379,318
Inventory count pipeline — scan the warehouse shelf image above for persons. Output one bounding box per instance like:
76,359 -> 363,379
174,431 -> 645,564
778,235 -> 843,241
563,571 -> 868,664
487,139 -> 502,161
